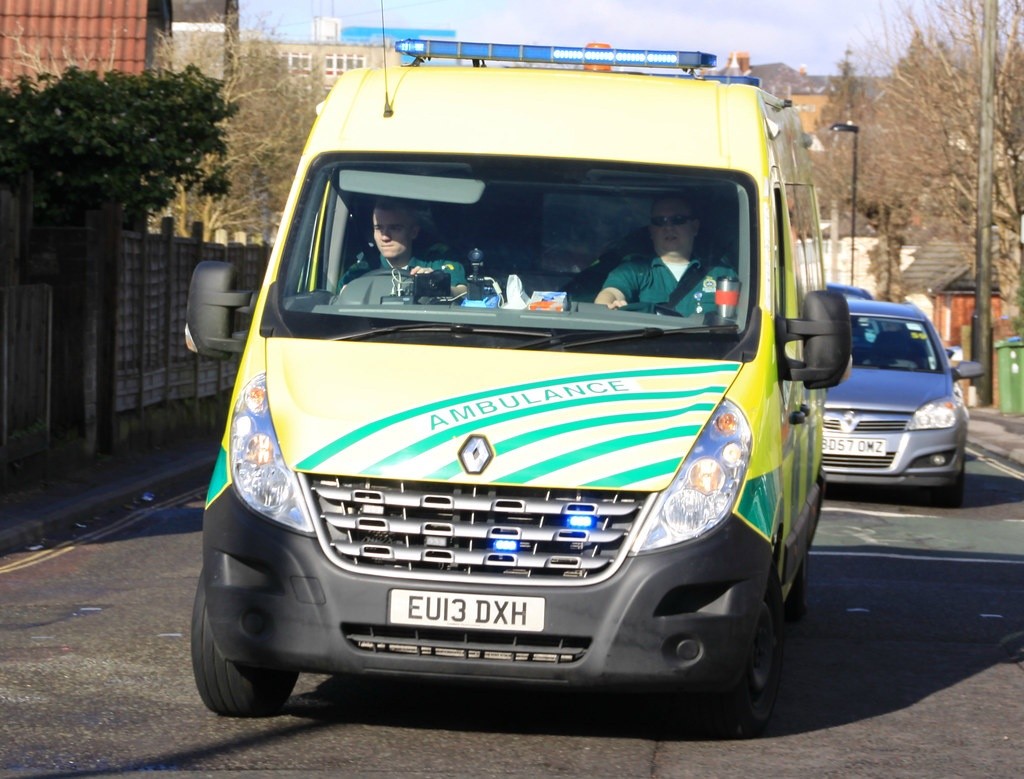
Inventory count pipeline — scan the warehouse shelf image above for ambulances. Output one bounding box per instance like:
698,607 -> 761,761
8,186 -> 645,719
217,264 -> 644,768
186,38 -> 824,735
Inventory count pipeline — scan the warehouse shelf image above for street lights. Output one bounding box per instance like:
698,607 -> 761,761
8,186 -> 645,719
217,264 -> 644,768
830,124 -> 859,288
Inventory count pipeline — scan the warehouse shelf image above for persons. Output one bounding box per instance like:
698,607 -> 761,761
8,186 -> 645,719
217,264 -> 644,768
335,198 -> 465,300
593,187 -> 743,313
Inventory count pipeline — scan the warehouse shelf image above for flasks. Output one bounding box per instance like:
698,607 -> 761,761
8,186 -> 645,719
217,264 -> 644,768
717,277 -> 740,318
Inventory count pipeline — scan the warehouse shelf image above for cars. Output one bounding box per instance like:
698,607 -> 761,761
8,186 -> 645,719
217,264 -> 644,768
819,298 -> 985,506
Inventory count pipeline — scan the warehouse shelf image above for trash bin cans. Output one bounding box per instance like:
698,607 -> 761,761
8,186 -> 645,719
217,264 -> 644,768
992,340 -> 1023,418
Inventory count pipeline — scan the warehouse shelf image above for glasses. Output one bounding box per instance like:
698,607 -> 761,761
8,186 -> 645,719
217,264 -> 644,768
649,211 -> 697,227
